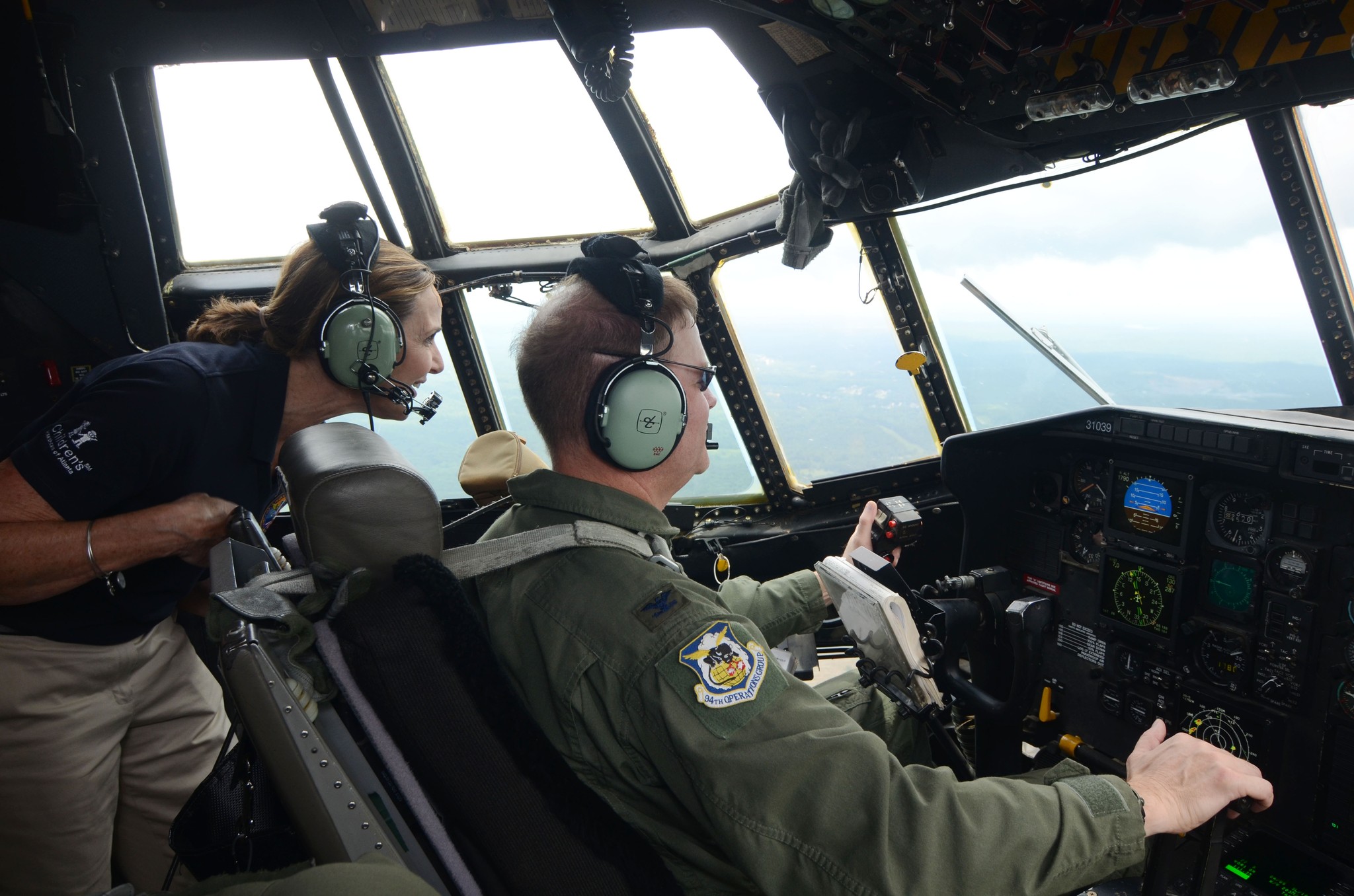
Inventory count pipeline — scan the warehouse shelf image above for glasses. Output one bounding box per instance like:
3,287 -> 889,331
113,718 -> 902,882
594,350 -> 717,391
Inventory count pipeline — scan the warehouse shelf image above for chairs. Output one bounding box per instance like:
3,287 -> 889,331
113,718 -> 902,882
210,423 -> 675,896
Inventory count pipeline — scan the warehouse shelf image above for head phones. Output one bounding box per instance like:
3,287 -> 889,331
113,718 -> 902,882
305,200 -> 407,392
565,233 -> 688,471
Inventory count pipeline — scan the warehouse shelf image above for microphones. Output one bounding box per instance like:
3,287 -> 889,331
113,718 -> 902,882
375,375 -> 444,425
704,423 -> 719,450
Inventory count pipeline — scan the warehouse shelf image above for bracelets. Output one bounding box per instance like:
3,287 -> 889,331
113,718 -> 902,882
87,519 -> 126,596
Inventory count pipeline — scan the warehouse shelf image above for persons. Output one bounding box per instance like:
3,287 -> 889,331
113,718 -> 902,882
473,271 -> 1275,896
0,236 -> 444,895
